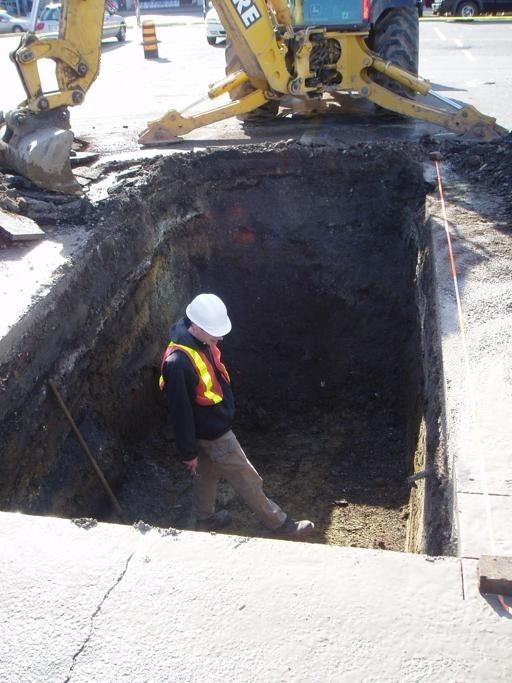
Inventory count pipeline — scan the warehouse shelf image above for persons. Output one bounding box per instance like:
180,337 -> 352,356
159,292 -> 316,539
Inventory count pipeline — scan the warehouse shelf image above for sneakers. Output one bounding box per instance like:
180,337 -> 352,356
195,509 -> 229,530
273,516 -> 314,536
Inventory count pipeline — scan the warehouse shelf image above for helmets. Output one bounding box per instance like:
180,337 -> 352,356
186,294 -> 232,337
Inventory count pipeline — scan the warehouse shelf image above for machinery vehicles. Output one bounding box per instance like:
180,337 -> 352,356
0,0 -> 510,203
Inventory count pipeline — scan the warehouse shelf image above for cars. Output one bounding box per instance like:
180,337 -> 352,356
427,0 -> 512,20
0,8 -> 31,33
201,3 -> 227,47
34,2 -> 128,43
